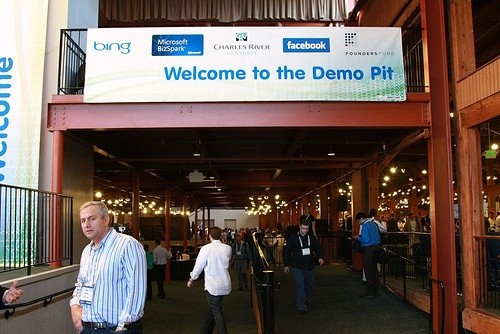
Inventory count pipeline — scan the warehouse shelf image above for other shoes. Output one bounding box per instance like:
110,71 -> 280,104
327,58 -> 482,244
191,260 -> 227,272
236,287 -> 243,291
156,295 -> 164,299
245,284 -> 247,288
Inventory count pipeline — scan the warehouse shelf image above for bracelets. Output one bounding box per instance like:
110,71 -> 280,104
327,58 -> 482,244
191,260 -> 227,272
190,278 -> 194,282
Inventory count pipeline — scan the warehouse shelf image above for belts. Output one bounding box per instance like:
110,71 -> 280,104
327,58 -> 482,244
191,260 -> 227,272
83,322 -> 117,329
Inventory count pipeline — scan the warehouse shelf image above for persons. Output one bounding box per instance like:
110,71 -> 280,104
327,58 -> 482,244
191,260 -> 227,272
282,218 -> 324,316
359,208 -> 386,285
189,223 -> 286,267
231,232 -> 252,290
187,226 -> 232,334
353,211 -> 381,300
143,244 -> 153,297
337,207 -> 500,265
153,239 -> 172,299
0,279 -> 23,307
299,205 -> 318,238
69,200 -> 147,333
